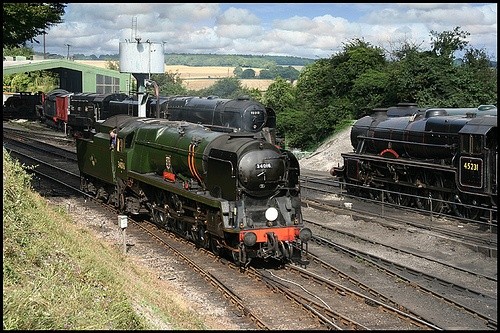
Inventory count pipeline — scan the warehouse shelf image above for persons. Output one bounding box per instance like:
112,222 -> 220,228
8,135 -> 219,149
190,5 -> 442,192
107,129 -> 119,150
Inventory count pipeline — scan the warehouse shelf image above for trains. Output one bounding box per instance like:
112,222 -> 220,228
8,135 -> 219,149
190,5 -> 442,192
4,91 -> 44,106
75,115 -> 311,274
36,88 -> 106,139
330,102 -> 499,227
102,80 -> 286,152
